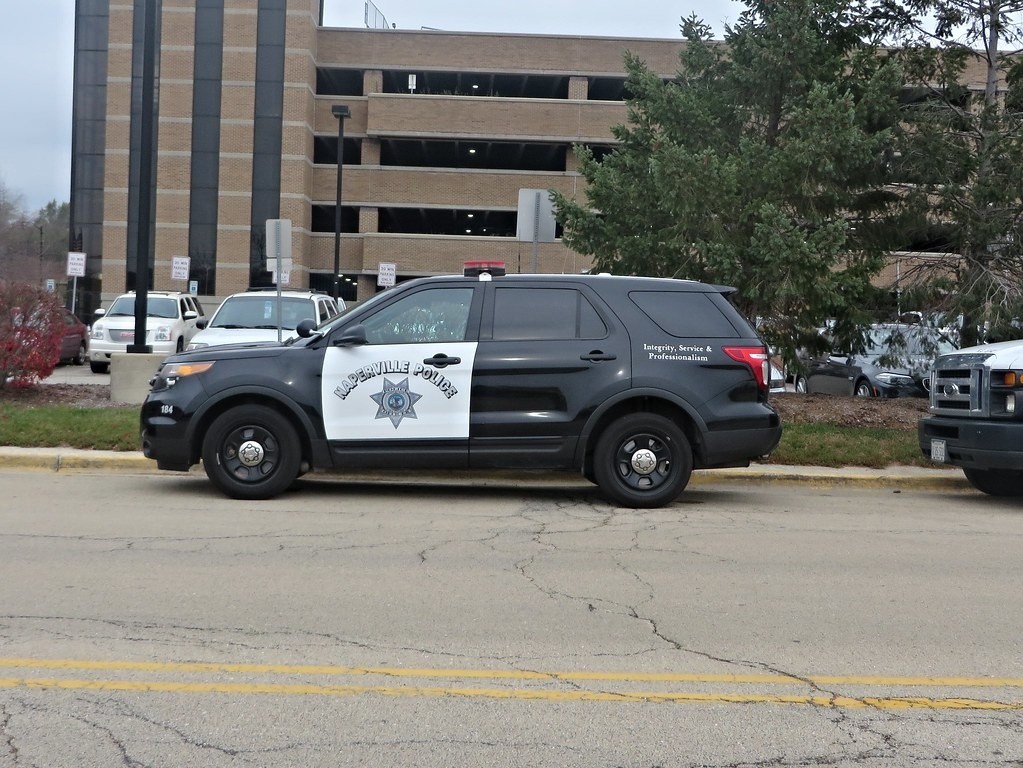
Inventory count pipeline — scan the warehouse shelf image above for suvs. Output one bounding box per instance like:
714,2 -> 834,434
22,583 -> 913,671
917,338 -> 1023,498
139,260 -> 781,509
86,292 -> 199,374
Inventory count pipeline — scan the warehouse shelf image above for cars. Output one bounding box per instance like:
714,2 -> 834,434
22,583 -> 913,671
1,305 -> 90,372
187,290 -> 342,348
761,313 -> 1019,397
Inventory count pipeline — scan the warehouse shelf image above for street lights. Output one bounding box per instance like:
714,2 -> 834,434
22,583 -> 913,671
331,104 -> 352,306
